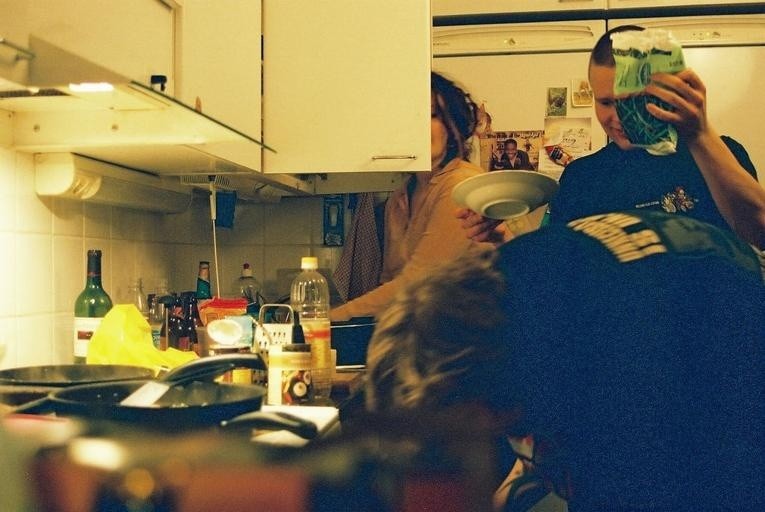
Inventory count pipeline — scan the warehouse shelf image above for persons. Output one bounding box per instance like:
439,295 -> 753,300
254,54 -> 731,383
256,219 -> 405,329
452,19 -> 764,258
489,136 -> 535,172
361,207 -> 764,512
319,69 -> 486,322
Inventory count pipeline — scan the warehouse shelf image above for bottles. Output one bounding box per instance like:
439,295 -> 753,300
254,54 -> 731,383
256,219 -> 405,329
130,259 -> 213,359
72,247 -> 113,367
291,256 -> 333,401
235,262 -> 261,302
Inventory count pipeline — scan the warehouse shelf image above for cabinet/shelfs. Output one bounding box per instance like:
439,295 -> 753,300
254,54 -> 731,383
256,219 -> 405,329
2,0 -> 438,195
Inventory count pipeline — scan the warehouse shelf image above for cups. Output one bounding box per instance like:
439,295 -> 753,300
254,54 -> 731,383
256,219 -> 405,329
266,352 -> 315,406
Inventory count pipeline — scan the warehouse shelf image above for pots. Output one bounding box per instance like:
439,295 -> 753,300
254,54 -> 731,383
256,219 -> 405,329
0,361 -> 156,418
52,351 -> 265,442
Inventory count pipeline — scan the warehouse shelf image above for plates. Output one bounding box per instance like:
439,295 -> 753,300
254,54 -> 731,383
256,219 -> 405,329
450,168 -> 558,222
